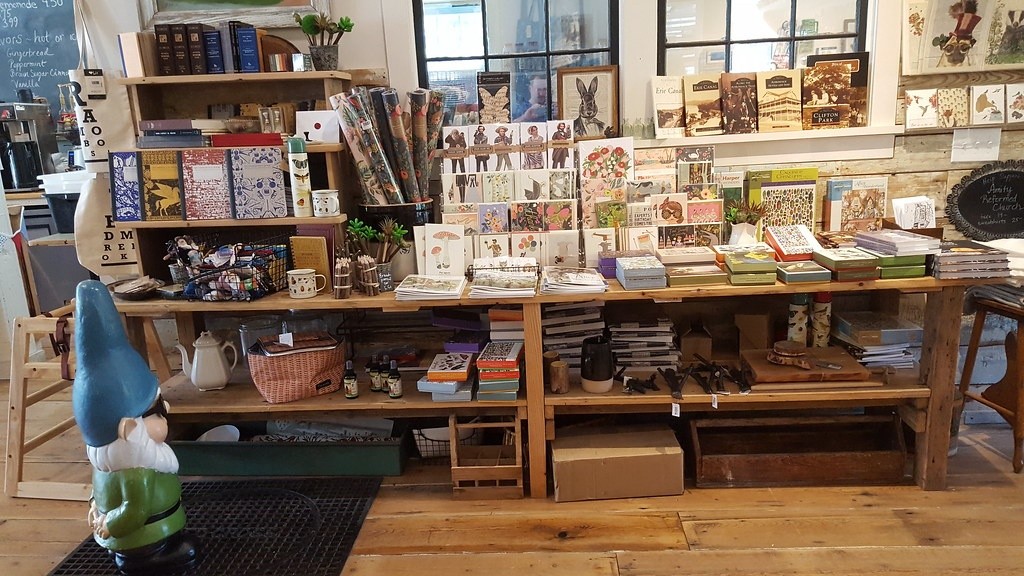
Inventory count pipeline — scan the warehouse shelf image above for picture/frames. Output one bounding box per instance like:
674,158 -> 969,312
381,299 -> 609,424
556,64 -> 620,142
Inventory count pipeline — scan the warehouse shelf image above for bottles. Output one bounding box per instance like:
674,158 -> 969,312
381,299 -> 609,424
811,291 -> 833,348
280,309 -> 327,335
343,361 -> 359,398
369,355 -> 402,399
287,138 -> 314,217
788,293 -> 809,349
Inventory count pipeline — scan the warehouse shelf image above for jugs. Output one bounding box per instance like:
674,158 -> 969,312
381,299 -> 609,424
8,142 -> 38,187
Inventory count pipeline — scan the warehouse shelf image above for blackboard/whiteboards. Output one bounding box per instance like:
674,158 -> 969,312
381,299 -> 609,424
0,0 -> 82,139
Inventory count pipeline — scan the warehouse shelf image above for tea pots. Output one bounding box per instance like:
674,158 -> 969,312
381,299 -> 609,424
175,331 -> 238,391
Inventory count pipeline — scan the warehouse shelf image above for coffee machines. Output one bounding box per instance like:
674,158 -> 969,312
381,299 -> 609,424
0,102 -> 60,193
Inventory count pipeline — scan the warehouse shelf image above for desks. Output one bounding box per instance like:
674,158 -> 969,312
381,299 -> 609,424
111,268 -> 1020,498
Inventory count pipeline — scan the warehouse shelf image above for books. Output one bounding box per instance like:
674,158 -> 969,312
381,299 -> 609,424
104,21 -> 1024,402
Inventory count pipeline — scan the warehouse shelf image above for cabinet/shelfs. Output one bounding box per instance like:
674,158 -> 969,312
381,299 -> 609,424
116,70 -> 353,280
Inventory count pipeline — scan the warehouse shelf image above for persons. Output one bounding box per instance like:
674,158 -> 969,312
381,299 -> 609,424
72,280 -> 195,576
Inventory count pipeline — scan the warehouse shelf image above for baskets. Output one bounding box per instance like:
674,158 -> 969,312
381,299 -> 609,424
165,229 -> 295,303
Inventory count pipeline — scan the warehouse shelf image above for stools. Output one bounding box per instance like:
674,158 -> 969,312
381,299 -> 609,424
946,293 -> 1024,473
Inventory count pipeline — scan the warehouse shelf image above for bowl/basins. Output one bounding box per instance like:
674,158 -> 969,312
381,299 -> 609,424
106,278 -> 165,300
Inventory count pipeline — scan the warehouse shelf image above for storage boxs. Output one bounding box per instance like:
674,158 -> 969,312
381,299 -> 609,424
37,168 -> 95,234
734,309 -> 772,358
552,418 -> 684,505
9,200 -> 30,267
831,307 -> 925,347
414,304 -> 526,402
595,239 -> 926,290
677,318 -> 713,361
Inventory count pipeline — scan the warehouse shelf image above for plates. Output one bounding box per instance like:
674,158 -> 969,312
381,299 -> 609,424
194,424 -> 242,442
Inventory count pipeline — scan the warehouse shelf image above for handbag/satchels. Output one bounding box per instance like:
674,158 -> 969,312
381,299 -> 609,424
73,173 -> 142,276
68,0 -> 136,173
246,330 -> 348,404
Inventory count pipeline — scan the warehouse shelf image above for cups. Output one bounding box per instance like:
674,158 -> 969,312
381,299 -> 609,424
581,336 -> 613,394
312,189 -> 341,217
551,361 -> 570,394
543,352 -> 560,384
287,269 -> 326,299
